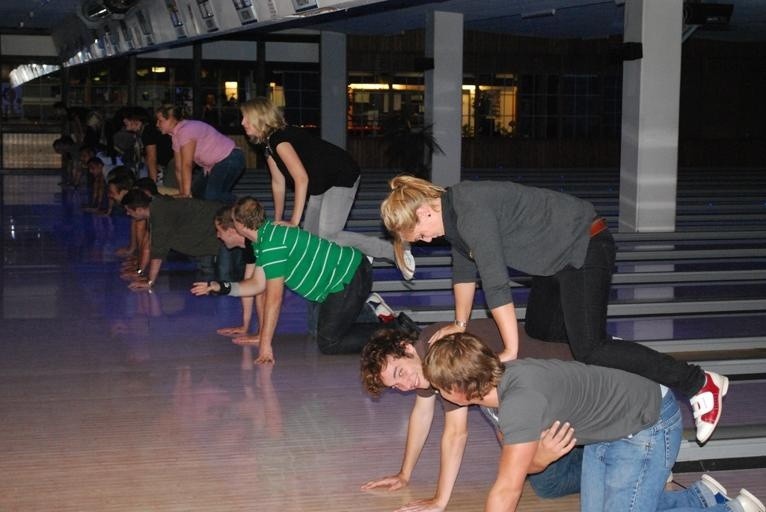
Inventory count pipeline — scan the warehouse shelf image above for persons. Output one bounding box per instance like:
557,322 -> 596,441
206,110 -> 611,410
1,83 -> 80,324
378,175 -> 727,449
192,200 -> 419,365
54,90 -> 419,343
361,327 -> 765,512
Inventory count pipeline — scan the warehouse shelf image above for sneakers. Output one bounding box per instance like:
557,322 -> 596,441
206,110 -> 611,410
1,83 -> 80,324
689,369 -> 730,444
396,312 -> 423,340
401,249 -> 416,281
736,488 -> 765,512
699,473 -> 732,505
365,292 -> 397,323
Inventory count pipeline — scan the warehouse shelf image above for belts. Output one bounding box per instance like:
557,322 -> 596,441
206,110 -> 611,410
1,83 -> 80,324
590,218 -> 609,236
